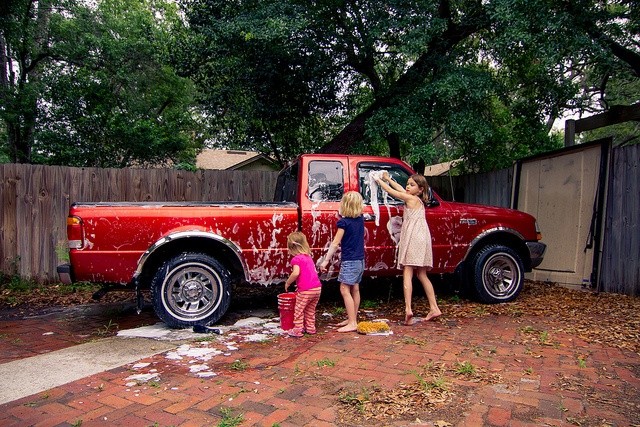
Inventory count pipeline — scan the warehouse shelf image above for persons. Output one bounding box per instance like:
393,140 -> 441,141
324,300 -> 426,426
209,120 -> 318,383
284,232 -> 322,336
319,191 -> 365,332
371,170 -> 442,325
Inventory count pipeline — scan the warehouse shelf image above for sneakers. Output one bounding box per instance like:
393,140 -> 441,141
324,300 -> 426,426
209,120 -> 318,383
288,328 -> 304,337
305,330 -> 316,334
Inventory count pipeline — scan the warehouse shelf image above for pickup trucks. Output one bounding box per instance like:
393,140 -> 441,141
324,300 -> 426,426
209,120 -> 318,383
67,152 -> 546,328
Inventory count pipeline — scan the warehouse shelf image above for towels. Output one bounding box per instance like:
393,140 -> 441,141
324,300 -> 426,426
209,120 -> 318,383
368,170 -> 391,226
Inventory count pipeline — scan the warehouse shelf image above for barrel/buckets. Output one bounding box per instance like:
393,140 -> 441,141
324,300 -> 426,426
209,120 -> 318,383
277,299 -> 297,304
277,291 -> 296,300
276,306 -> 296,331
278,302 -> 296,307
